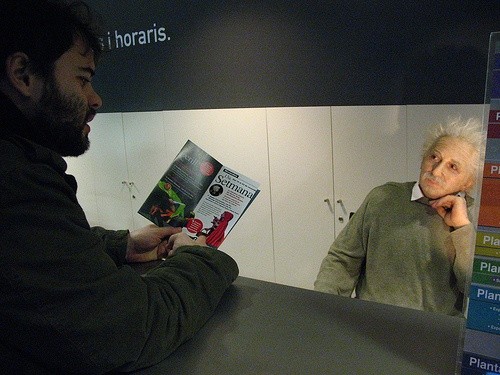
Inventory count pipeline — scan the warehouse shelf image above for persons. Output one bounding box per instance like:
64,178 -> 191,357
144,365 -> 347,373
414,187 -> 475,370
0,0 -> 240,375
314,114 -> 487,318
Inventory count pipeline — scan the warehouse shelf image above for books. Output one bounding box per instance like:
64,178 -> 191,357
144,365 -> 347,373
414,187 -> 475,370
138,140 -> 261,250
